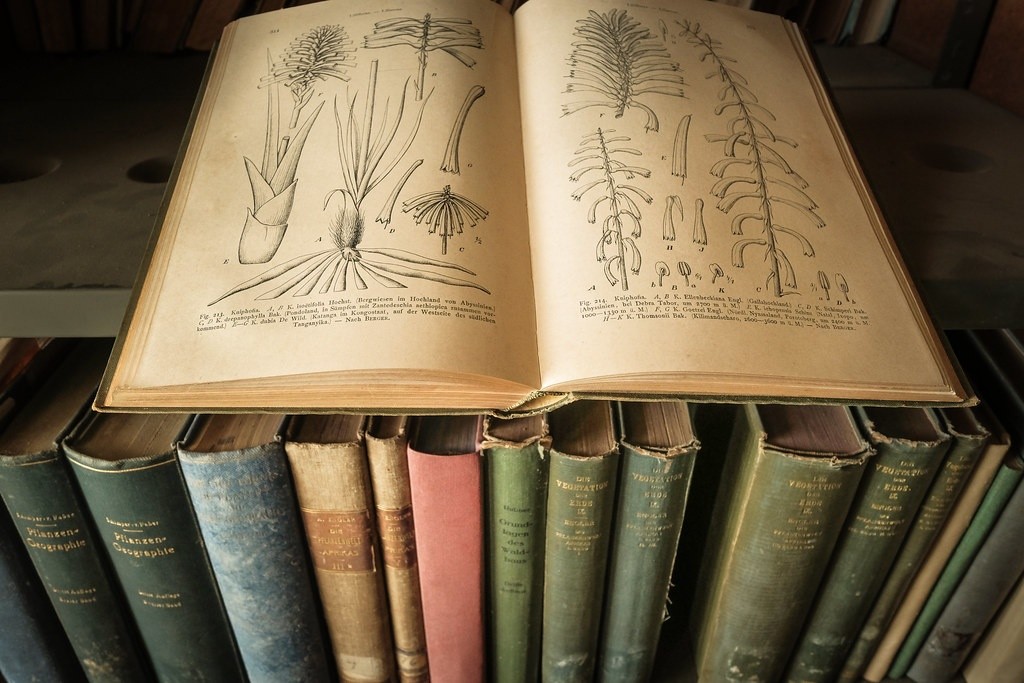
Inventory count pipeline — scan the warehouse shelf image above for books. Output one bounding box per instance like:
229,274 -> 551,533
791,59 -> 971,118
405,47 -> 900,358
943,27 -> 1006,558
0,0 -> 902,93
93,0 -> 981,419
0,338 -> 1024,683
